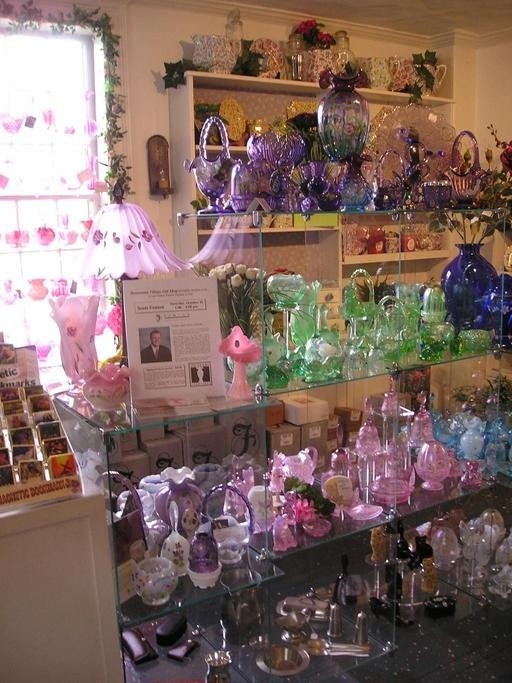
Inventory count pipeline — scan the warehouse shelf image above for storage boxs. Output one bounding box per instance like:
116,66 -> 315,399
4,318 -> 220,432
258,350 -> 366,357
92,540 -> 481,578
95,395 -> 333,493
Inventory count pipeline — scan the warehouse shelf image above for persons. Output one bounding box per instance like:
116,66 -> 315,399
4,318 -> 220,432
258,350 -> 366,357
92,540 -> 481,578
140,329 -> 171,361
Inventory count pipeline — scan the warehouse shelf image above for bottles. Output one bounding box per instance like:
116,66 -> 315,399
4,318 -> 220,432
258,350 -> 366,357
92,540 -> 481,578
333,30 -> 349,48
283,33 -> 310,81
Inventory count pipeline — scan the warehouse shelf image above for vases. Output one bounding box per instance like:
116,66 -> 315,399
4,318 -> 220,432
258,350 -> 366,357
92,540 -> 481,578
228,340 -> 269,378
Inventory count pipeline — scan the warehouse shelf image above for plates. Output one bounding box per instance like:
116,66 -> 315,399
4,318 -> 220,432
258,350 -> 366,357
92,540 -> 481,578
252,38 -> 284,79
373,103 -> 463,194
218,99 -> 246,141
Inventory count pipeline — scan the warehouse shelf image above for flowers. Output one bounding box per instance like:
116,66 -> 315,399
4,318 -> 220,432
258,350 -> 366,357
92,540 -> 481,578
200,262 -> 265,335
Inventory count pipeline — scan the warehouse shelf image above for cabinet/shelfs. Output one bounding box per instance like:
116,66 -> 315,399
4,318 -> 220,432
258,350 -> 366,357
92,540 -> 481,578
51,212 -> 509,679
165,69 -> 452,409
1,497 -> 126,683
